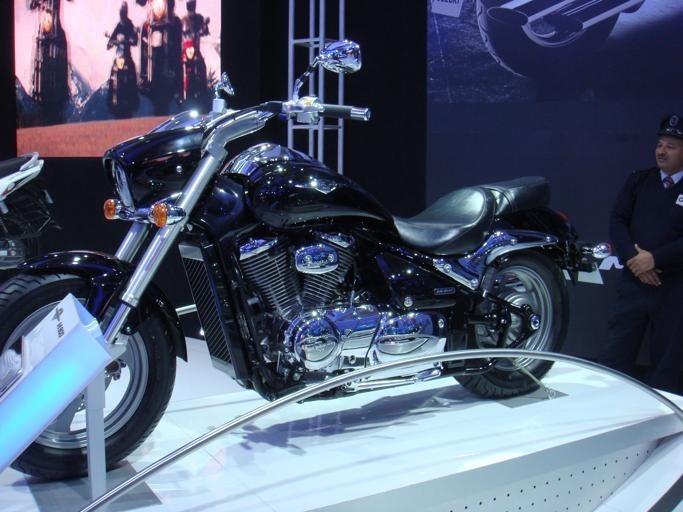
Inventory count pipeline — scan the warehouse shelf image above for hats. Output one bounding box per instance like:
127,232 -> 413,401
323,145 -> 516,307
655,111 -> 683,140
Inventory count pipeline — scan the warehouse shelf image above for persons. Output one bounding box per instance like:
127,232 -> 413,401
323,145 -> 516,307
106,0 -> 210,93
603,114 -> 682,396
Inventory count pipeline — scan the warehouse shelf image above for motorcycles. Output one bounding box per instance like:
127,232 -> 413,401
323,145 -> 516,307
1,41 -> 611,481
109,0 -> 207,118
29,0 -> 68,116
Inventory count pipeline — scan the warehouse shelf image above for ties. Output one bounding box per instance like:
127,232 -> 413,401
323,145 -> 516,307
662,174 -> 674,190
